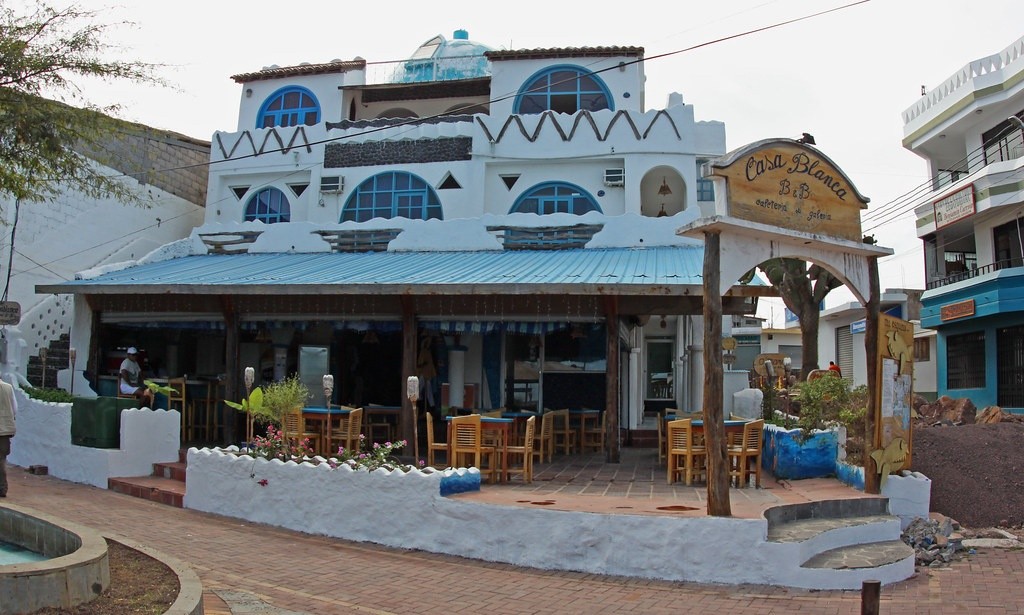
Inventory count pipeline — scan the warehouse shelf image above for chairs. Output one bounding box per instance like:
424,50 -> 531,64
668,418 -> 705,486
281,402 -> 607,484
726,419 -> 764,488
657,413 -> 666,464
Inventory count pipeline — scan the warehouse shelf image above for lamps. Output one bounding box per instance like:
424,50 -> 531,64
620,62 -> 626,72
976,110 -> 982,114
658,177 -> 672,196
246,89 -> 252,97
939,134 -> 946,138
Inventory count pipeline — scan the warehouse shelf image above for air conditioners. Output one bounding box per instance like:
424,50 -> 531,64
320,175 -> 344,194
603,168 -> 625,187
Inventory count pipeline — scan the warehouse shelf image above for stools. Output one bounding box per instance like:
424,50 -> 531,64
168,377 -> 193,444
195,380 -> 219,442
118,374 -> 137,400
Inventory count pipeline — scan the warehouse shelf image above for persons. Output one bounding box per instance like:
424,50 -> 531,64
829,361 -> 842,379
0,370 -> 19,497
119,347 -> 156,408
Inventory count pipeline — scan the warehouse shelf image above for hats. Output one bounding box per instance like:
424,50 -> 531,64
127,347 -> 137,354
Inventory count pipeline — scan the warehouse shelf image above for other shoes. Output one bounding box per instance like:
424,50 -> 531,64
0,493 -> 6,497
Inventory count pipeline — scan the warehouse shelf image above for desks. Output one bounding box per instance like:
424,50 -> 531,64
364,407 -> 402,447
691,420 -> 749,483
502,412 -> 544,464
446,415 -> 515,483
569,409 -> 598,456
301,408 -> 351,458
